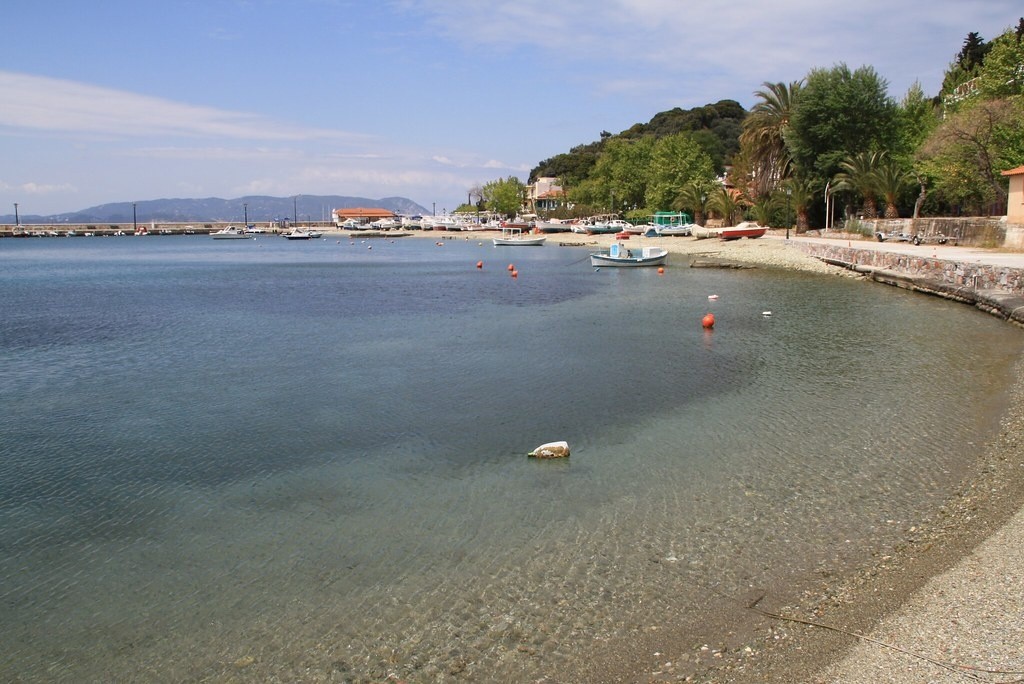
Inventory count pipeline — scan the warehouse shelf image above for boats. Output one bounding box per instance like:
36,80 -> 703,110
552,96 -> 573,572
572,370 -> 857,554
333,213 -> 653,236
492,226 -> 547,246
209,225 -> 250,239
12,225 -> 172,237
589,242 -> 669,267
284,228 -> 323,240
690,221 -> 770,240
642,213 -> 691,237
